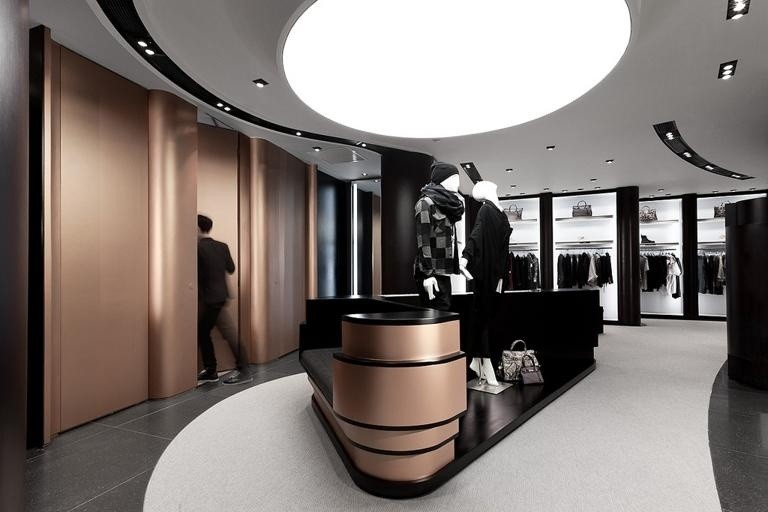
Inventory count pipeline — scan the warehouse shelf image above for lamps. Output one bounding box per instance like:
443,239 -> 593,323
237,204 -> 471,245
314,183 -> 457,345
275,0 -> 632,139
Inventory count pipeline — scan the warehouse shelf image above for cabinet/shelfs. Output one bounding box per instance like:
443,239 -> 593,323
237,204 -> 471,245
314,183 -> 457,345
697,217 -> 725,246
641,219 -> 679,245
507,219 -> 538,245
555,215 -> 613,244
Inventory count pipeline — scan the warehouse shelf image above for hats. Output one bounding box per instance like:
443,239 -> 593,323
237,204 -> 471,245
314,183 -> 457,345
429,161 -> 460,186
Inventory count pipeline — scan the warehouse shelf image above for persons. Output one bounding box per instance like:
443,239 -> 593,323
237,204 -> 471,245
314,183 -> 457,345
198,214 -> 255,385
414,161 -> 464,310
458,180 -> 513,387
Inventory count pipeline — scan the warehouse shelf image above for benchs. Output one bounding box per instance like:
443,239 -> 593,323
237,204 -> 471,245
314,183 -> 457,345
299,295 -> 467,500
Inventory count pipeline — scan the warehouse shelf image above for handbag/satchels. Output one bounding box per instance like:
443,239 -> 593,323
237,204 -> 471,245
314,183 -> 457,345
503,203 -> 525,222
639,205 -> 658,224
495,338 -> 542,384
519,354 -> 546,385
572,200 -> 593,218
713,200 -> 732,218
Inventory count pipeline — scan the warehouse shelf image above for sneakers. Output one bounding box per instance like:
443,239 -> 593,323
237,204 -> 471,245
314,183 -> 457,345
222,369 -> 254,386
197,369 -> 220,383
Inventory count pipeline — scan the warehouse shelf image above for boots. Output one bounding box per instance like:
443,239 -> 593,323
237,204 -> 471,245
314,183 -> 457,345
641,234 -> 655,244
